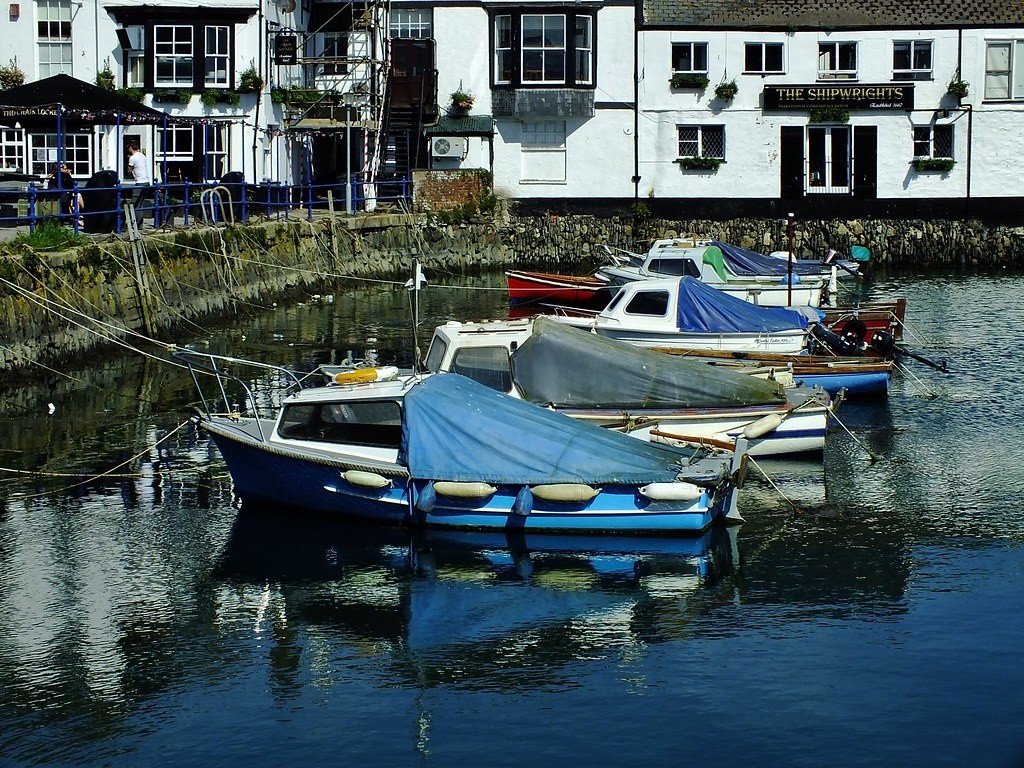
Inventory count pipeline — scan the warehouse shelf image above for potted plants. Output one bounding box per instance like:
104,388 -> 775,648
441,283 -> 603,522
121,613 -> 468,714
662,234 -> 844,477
678,154 -> 720,172
908,157 -> 955,172
948,79 -> 969,98
810,109 -> 849,122
93,55 -> 343,107
669,74 -> 737,101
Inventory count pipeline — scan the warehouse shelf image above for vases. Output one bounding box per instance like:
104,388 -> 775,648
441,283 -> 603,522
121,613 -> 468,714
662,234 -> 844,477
447,107 -> 470,118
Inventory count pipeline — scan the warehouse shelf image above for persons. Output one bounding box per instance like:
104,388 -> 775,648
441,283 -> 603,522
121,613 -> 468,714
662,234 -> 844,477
43,160 -> 85,225
126,139 -> 150,229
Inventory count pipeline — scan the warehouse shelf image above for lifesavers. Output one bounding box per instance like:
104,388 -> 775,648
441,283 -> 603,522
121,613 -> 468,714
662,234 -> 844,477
331,365 -> 400,385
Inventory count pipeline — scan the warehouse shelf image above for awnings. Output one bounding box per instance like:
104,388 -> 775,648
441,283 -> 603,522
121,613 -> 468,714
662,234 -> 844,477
102,1 -> 260,24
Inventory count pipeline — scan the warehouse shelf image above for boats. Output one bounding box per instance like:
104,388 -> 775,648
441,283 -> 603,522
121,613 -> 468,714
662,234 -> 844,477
531,279 -> 825,360
505,265 -> 601,296
596,244 -> 861,298
196,365 -> 739,535
323,322 -> 833,458
819,305 -> 907,350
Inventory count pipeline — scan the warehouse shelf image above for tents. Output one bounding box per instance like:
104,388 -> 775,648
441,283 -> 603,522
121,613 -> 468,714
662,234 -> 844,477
0,73 -> 176,166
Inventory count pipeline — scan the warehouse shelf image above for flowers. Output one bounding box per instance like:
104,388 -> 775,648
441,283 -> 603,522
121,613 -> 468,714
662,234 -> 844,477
452,92 -> 472,110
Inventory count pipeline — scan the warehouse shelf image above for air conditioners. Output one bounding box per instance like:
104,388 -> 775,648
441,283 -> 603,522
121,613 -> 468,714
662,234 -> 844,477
432,137 -> 463,157
